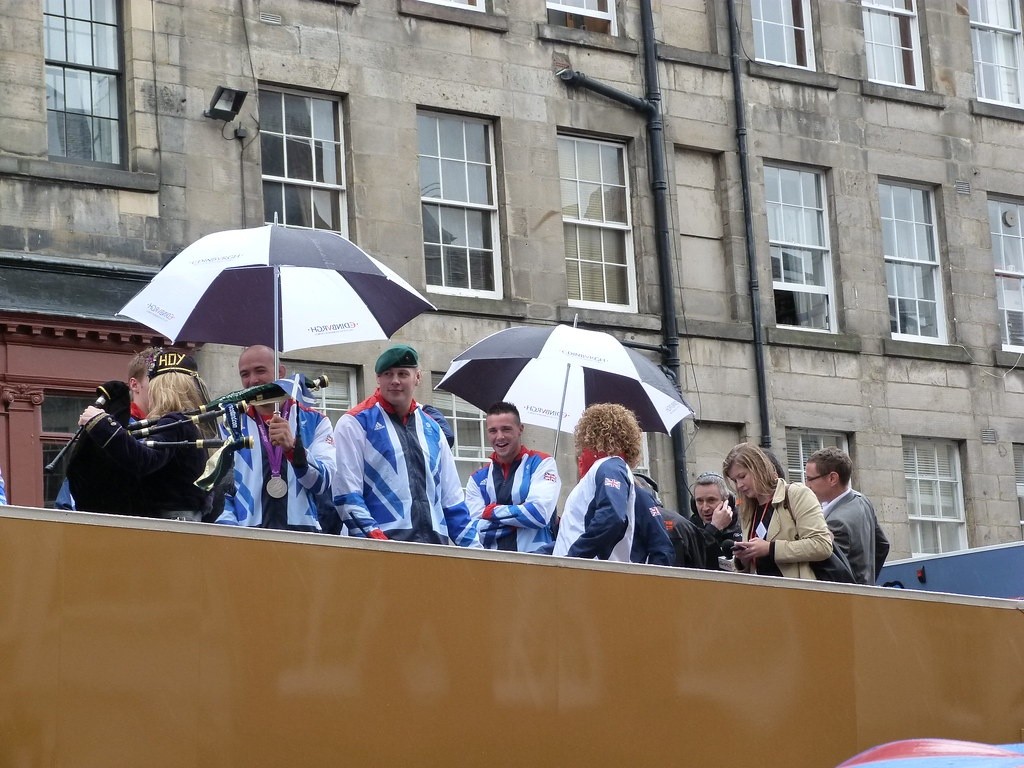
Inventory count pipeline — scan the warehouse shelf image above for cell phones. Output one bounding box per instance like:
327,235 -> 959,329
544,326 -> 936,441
730,545 -> 746,551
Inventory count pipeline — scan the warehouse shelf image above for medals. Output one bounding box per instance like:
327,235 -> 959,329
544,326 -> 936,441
266,476 -> 288,499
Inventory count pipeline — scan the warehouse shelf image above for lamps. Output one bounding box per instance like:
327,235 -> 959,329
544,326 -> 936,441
204,85 -> 248,122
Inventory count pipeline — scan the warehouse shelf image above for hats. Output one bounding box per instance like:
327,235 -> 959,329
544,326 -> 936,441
633,472 -> 658,491
147,348 -> 198,381
374,344 -> 419,375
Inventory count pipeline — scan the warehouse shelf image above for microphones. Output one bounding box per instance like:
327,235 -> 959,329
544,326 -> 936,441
681,469 -> 693,498
717,539 -> 738,573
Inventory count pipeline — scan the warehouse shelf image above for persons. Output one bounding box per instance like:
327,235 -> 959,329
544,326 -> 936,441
213,345 -> 336,535
57,345 -> 233,526
464,402 -> 562,558
633,431 -> 890,585
333,346 -> 473,552
550,405 -> 639,562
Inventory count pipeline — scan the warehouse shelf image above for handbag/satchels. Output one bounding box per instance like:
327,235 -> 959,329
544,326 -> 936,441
810,540 -> 856,583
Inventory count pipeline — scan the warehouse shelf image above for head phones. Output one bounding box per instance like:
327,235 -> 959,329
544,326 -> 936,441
688,474 -> 736,515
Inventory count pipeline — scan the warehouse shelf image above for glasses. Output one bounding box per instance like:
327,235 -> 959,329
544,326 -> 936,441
805,472 -> 830,485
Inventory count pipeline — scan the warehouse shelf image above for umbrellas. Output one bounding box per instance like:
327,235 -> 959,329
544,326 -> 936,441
113,213 -> 439,416
429,311 -> 691,457
